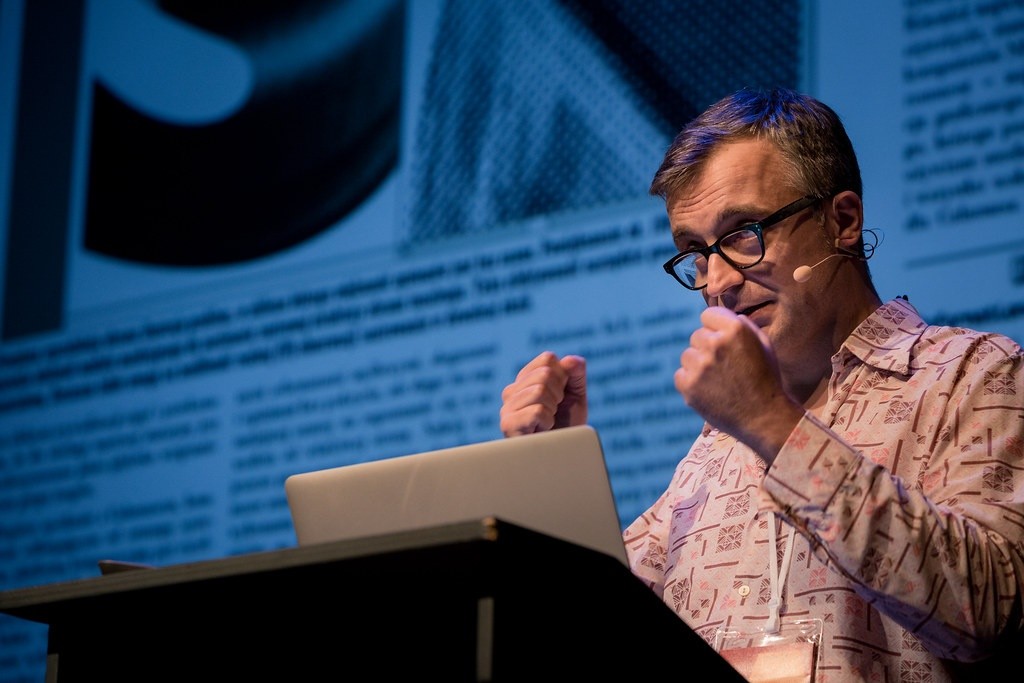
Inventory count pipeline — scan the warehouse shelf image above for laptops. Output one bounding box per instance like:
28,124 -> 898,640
285,424 -> 630,571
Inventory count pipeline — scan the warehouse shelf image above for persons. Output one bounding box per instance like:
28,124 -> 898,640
499,87 -> 1024,683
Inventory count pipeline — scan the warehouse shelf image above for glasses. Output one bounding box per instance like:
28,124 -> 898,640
663,190 -> 837,290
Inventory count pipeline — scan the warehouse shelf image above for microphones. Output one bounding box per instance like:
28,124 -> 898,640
794,254 -> 853,284
